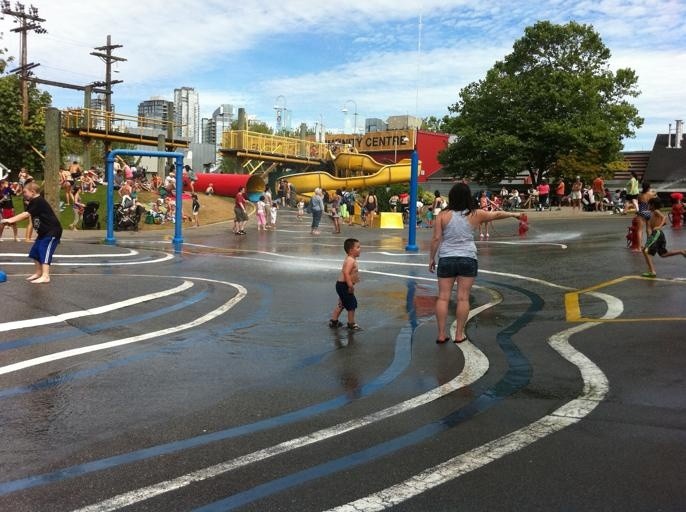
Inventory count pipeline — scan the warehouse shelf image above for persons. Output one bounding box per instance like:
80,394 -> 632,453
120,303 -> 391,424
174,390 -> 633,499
429,183 -> 521,343
329,238 -> 361,330
0,183 -> 62,283
641,198 -> 686,277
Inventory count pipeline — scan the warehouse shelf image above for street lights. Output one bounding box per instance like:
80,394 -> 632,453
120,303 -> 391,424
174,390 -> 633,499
341,97 -> 357,152
315,112 -> 324,144
393,125 -> 410,163
273,93 -> 289,137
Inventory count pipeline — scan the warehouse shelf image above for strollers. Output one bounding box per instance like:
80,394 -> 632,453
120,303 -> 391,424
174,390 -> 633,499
110,202 -> 144,232
79,200 -> 101,231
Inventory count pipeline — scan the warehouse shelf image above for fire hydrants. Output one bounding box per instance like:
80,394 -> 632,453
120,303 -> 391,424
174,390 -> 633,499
668,202 -> 684,228
516,212 -> 531,237
624,213 -> 646,253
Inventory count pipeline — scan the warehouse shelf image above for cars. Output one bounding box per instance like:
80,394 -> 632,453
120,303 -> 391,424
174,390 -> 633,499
504,195 -> 535,208
562,189 -> 590,207
473,190 -> 498,210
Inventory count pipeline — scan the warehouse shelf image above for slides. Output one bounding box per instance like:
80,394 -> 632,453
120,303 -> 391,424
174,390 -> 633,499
276,147 -> 422,229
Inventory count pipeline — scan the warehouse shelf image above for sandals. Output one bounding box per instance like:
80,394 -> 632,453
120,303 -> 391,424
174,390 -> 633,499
452,333 -> 470,344
433,332 -> 450,345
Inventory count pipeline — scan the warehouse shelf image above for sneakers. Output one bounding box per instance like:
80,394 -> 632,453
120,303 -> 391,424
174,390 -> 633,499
346,321 -> 360,333
641,269 -> 657,279
238,229 -> 248,235
329,318 -> 344,331
